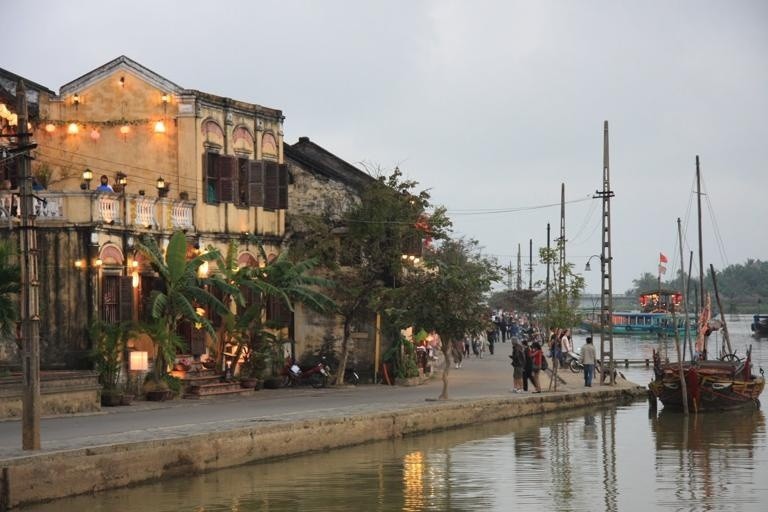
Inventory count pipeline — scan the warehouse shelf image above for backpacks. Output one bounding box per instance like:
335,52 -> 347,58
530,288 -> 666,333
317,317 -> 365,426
541,355 -> 547,371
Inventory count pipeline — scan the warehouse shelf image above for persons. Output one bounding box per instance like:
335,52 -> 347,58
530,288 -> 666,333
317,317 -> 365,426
449,303 -> 571,393
32,176 -> 44,191
0,180 -> 20,218
96,173 -> 113,192
578,338 -> 596,388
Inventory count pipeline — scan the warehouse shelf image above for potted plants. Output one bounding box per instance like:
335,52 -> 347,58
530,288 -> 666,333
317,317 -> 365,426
143,318 -> 186,402
241,323 -> 290,393
84,316 -> 137,407
400,366 -> 420,385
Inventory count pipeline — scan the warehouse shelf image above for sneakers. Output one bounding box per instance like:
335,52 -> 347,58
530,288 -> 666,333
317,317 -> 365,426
509,388 -> 523,395
454,363 -> 461,369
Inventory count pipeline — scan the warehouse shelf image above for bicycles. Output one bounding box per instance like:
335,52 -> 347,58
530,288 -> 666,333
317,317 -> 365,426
478,335 -> 486,359
531,332 -> 544,347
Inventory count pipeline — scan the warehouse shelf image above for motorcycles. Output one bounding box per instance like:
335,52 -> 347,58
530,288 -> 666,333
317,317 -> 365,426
281,343 -> 360,388
567,351 -> 601,373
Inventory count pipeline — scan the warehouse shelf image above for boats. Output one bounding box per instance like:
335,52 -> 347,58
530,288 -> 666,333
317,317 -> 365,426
648,155 -> 766,410
751,315 -> 768,333
577,287 -> 718,336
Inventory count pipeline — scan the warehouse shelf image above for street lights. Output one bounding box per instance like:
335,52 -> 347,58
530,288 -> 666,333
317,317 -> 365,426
584,254 -> 605,385
117,172 -> 133,325
